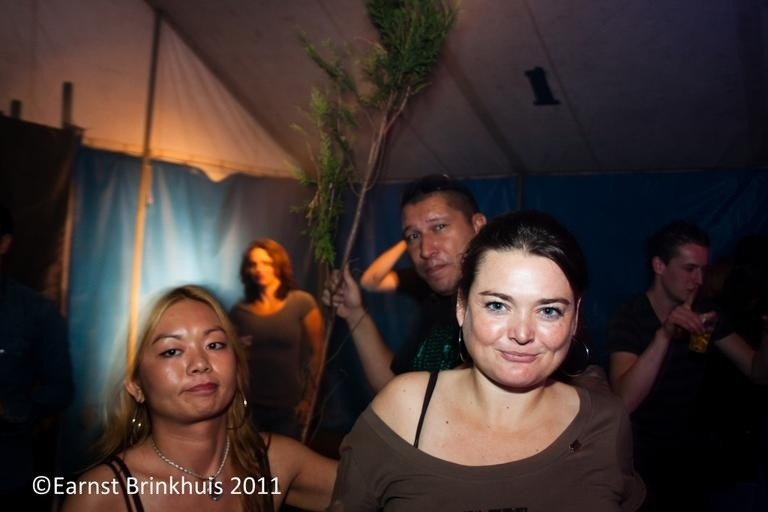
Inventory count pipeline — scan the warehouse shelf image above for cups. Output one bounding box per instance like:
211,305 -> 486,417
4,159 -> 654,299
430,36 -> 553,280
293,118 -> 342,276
688,313 -> 713,353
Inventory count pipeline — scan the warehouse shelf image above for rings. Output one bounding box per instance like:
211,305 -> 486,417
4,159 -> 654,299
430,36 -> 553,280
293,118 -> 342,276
682,302 -> 690,312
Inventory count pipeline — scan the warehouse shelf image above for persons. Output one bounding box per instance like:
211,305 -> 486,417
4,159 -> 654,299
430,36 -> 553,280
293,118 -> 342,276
606,222 -> 766,510
64,286 -> 339,511
321,174 -> 615,397
361,237 -> 435,302
0,201 -> 77,512
328,211 -> 645,511
228,239 -> 326,434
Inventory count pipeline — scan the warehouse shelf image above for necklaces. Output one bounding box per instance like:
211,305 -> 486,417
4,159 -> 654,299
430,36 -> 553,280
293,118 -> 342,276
150,432 -> 230,500
